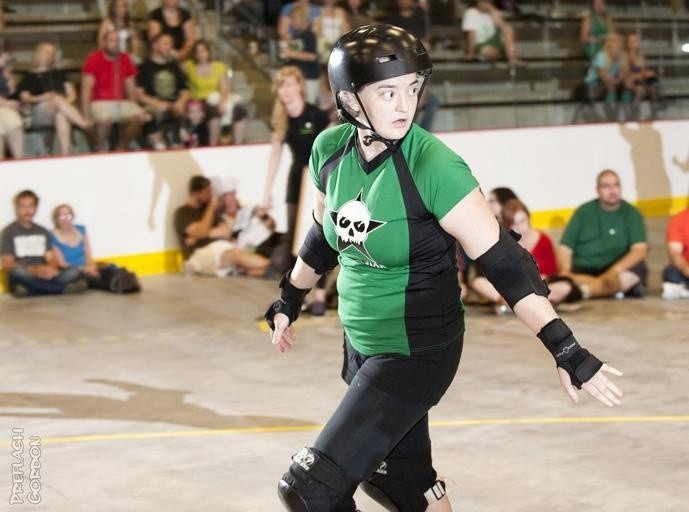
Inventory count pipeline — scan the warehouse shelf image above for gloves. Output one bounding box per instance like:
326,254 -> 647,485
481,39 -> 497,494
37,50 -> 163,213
536,318 -> 603,390
263,268 -> 312,333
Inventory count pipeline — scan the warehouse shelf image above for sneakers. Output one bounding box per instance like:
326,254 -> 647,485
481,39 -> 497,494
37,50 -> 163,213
64,279 -> 88,294
15,282 -> 29,297
660,282 -> 688,301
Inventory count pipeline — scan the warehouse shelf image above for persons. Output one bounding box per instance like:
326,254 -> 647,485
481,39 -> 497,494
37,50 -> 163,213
256,52 -> 342,317
172,173 -> 272,278
264,22 -> 625,512
660,204 -> 689,298
559,167 -> 653,301
0,186 -> 88,296
469,197 -> 557,307
483,185 -> 523,219
1,1 -> 689,160
218,178 -> 292,278
47,199 -> 146,294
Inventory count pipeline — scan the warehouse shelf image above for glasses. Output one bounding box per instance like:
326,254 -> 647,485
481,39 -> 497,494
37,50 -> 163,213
56,212 -> 73,221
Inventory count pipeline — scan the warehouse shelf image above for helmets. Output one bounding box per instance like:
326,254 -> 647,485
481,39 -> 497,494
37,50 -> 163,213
327,23 -> 434,110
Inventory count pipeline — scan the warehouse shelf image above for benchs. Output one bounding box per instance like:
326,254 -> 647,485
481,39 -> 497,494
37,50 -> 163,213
417,1 -> 688,129
1,1 -> 144,74
